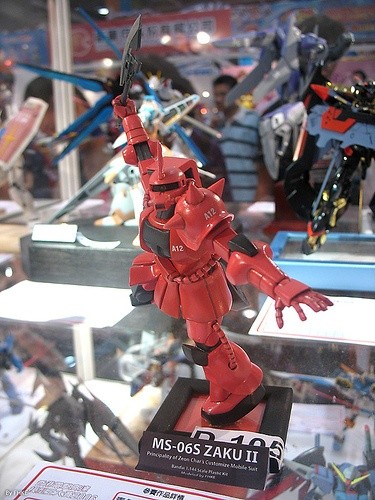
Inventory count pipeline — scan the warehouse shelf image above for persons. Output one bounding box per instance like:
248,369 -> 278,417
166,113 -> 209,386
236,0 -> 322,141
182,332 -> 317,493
1,68 -> 374,237
